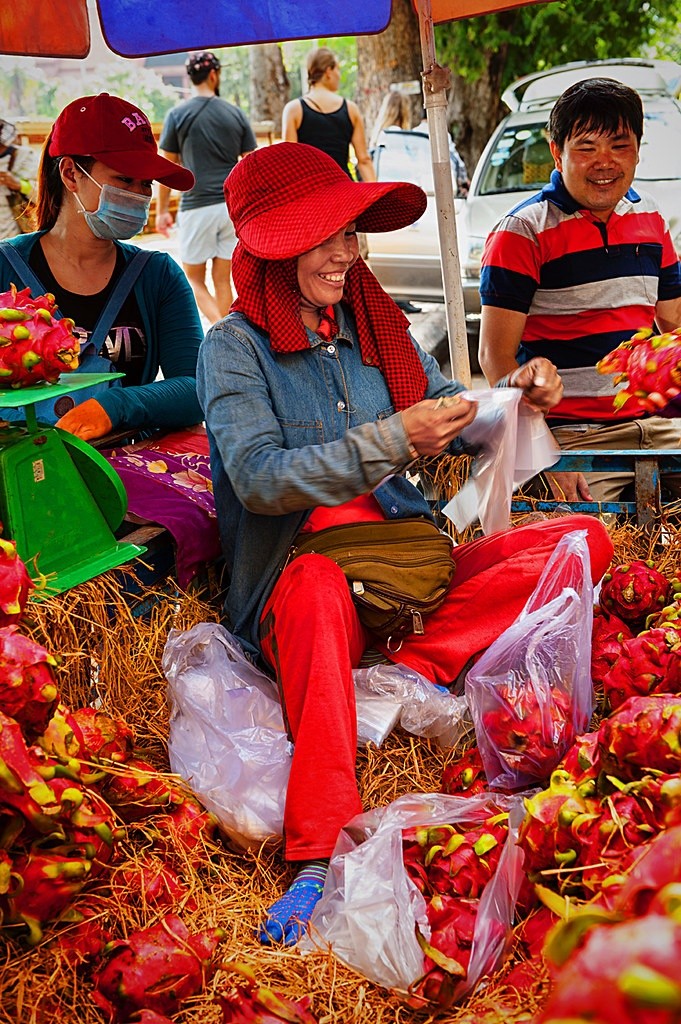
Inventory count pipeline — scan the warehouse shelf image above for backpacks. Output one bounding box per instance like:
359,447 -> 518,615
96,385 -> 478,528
0,238 -> 158,443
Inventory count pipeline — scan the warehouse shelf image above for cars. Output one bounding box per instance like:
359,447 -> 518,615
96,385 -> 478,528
349,57 -> 681,314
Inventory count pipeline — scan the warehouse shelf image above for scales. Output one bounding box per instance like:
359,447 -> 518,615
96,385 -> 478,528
0,373 -> 148,602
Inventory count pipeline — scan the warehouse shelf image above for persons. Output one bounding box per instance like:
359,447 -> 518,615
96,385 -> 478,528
351,87 -> 418,201
153,50 -> 259,325
280,47 -> 379,183
0,91 -> 214,592
195,142 -> 615,945
410,89 -> 471,200
476,76 -> 681,541
0,119 -> 40,239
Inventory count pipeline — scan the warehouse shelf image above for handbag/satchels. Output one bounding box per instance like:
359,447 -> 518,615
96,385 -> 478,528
461,526 -> 594,790
160,619 -> 309,845
297,782 -> 524,1006
281,520 -> 456,651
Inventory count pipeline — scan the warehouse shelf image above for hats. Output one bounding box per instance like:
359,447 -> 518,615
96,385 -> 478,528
48,91 -> 194,192
223,143 -> 432,261
183,51 -> 220,74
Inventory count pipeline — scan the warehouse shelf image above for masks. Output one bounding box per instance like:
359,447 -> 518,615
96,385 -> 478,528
67,165 -> 152,243
